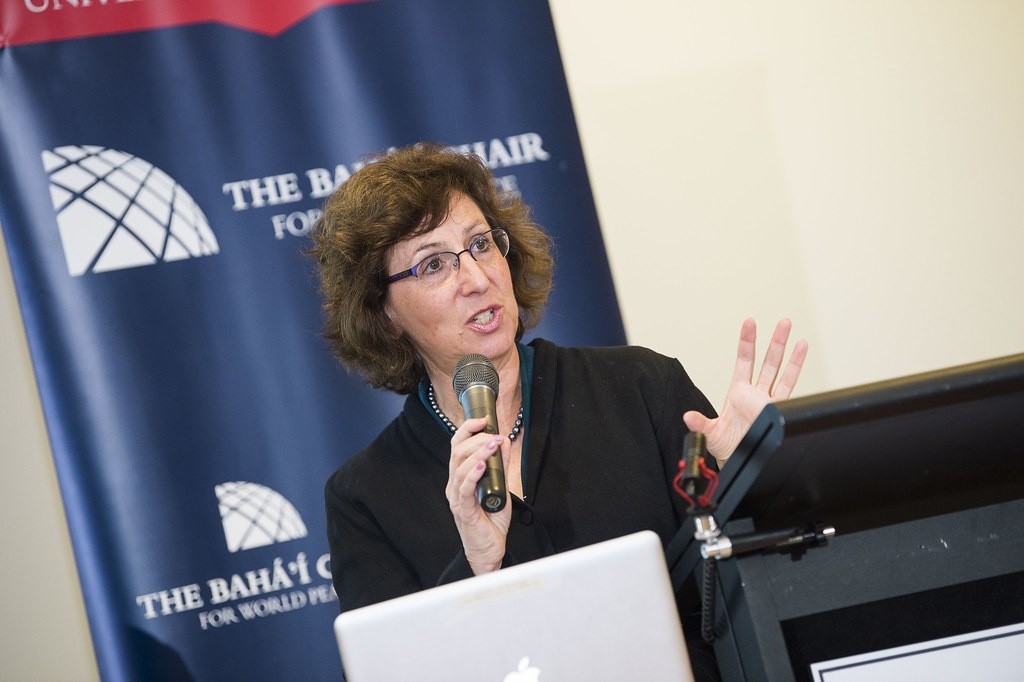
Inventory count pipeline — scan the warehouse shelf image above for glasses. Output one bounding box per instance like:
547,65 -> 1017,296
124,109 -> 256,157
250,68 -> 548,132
385,227 -> 509,287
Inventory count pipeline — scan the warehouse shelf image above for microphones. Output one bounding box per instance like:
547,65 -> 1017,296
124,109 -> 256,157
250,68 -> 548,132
682,432 -> 707,500
453,353 -> 507,513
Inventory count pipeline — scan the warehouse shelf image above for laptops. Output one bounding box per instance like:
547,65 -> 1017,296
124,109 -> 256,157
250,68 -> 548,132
334,529 -> 695,682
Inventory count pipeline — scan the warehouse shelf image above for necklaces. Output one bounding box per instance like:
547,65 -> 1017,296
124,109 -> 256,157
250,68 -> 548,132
427,383 -> 524,442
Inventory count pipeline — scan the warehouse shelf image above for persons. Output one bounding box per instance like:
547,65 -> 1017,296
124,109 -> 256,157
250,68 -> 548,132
303,146 -> 808,682
